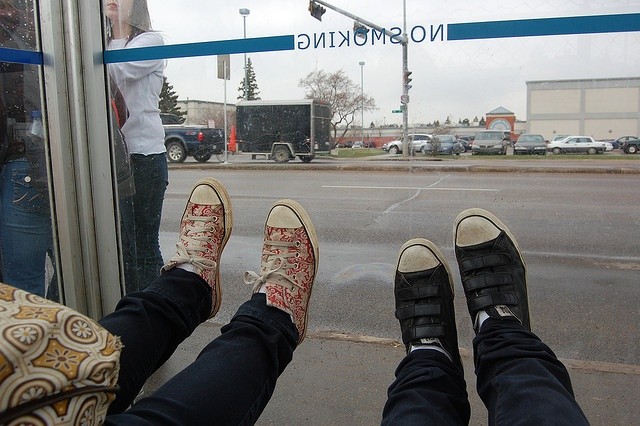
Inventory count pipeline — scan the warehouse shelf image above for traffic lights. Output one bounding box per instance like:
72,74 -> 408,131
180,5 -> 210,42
355,24 -> 369,34
314,4 -> 326,14
406,71 -> 412,88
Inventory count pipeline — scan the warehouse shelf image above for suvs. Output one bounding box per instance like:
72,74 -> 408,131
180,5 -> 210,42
382,133 -> 433,154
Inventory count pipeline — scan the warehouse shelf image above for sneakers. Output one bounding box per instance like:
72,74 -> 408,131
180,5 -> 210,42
244,198 -> 320,344
160,177 -> 233,318
452,206 -> 531,340
394,237 -> 464,381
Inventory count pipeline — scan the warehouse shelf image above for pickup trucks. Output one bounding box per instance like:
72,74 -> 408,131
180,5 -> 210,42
165,127 -> 225,162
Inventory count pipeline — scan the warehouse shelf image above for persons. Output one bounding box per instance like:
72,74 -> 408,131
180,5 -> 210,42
382,207 -> 589,425
0,0 -> 62,305
99,0 -> 168,293
92,178 -> 320,424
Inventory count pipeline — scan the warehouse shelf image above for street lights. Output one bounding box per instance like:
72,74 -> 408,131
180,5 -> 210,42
359,61 -> 365,147
239,8 -> 250,100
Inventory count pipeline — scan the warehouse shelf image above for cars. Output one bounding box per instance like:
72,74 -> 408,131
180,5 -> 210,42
513,133 -> 547,154
458,138 -> 472,152
425,134 -> 461,154
334,139 -> 375,147
472,130 -> 509,154
547,134 -> 640,154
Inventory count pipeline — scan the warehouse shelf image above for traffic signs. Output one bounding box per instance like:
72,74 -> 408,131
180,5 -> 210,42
393,110 -> 402,112
